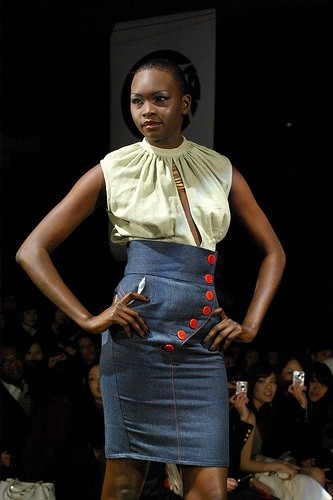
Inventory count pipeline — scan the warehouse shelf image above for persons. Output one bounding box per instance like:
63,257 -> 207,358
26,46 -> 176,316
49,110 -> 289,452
15,50 -> 286,500
0,293 -> 333,500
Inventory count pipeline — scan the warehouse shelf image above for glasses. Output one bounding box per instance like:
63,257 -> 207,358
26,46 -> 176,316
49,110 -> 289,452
0,356 -> 24,366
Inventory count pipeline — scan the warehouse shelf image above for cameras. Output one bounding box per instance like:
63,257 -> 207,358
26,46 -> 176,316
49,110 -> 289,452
236,381 -> 248,395
293,371 -> 305,387
